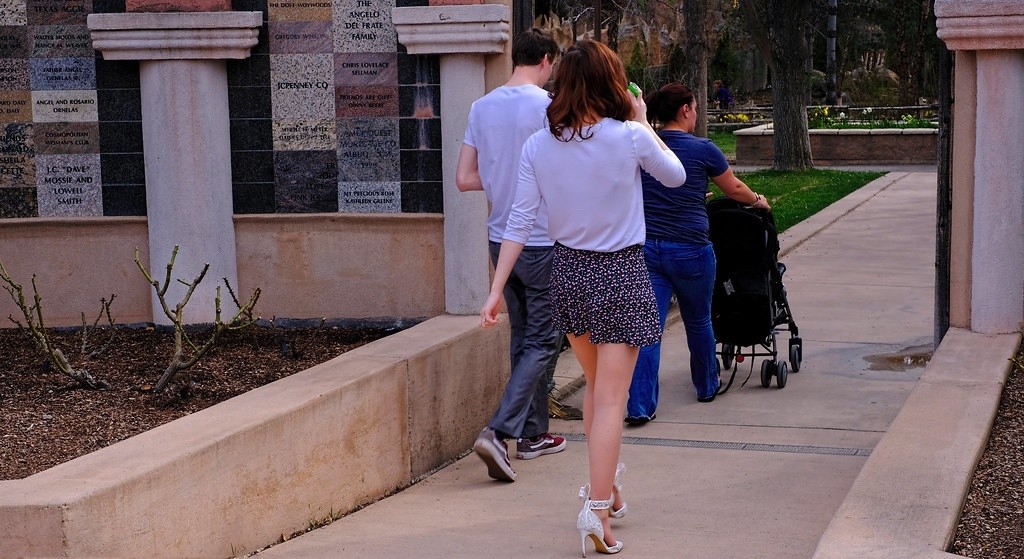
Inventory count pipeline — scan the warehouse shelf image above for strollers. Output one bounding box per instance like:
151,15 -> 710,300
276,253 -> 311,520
706,192 -> 802,396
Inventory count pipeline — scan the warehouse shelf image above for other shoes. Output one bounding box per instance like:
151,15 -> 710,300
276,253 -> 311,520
697,380 -> 723,403
624,414 -> 657,426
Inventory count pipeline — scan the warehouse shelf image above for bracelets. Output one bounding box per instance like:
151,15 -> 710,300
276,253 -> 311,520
752,192 -> 760,205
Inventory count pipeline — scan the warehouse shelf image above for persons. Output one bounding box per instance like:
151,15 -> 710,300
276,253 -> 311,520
456,30 -> 567,484
477,38 -> 687,555
622,82 -> 773,428
713,80 -> 734,110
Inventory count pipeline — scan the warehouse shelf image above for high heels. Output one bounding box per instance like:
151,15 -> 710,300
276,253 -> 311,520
576,500 -> 623,557
579,463 -> 627,519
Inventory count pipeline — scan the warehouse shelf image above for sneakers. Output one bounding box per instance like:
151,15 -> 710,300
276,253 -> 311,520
516,432 -> 566,459
473,427 -> 517,482
